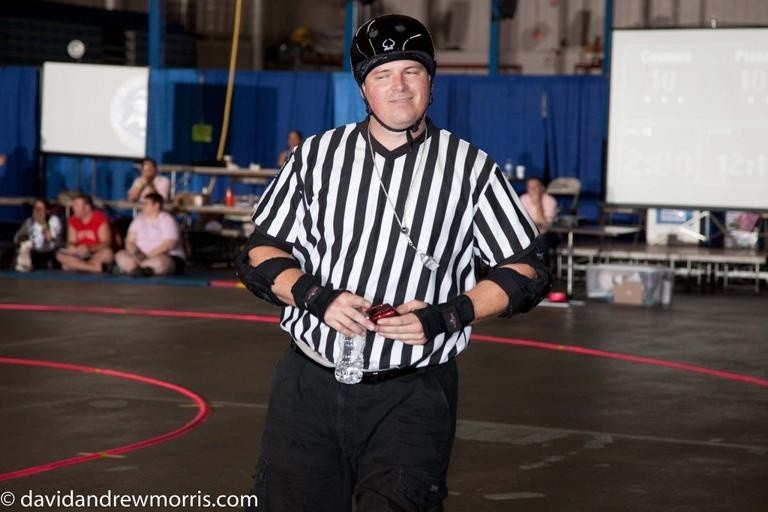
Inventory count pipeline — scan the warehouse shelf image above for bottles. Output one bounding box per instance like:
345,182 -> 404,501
333,308 -> 365,384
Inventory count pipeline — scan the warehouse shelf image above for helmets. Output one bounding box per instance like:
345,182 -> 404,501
350,14 -> 436,87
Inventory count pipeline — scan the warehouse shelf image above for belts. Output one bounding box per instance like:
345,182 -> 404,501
288,380 -> 296,382
290,340 -> 413,384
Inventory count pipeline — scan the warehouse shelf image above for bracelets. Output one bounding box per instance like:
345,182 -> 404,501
135,179 -> 146,190
41,221 -> 49,231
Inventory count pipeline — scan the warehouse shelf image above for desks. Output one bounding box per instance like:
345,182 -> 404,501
549,224 -> 642,297
0,198 -> 258,272
133,164 -> 278,201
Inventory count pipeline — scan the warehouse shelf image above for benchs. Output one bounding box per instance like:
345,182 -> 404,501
557,244 -> 768,294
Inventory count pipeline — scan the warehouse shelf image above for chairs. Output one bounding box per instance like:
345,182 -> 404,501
547,177 -> 581,226
598,209 -> 645,244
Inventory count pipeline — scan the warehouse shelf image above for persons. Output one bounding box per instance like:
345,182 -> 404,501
127,158 -> 172,204
114,190 -> 186,278
55,191 -> 117,276
520,175 -> 558,252
11,198 -> 63,272
233,12 -> 550,511
278,130 -> 303,169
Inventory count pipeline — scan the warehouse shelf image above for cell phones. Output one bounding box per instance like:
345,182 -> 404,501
367,303 -> 400,325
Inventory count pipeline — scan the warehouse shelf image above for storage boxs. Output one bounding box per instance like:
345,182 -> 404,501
586,263 -> 673,306
